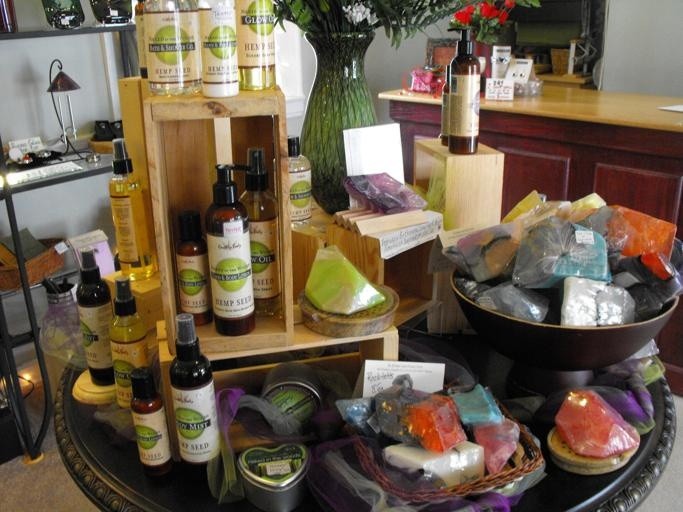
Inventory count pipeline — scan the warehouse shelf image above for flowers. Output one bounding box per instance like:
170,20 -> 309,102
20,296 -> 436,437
449,0 -> 516,44
273,2 -> 542,50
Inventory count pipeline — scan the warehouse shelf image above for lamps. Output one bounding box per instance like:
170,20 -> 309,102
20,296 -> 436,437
43,59 -> 84,165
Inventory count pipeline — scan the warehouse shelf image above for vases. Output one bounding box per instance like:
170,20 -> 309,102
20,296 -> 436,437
299,31 -> 378,214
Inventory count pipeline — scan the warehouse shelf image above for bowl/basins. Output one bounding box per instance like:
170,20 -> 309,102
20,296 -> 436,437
451,242 -> 680,369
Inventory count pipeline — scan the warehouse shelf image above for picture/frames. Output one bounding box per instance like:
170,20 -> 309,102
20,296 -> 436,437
426,37 -> 459,77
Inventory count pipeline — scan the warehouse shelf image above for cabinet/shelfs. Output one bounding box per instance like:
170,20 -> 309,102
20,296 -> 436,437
379,83 -> 683,395
0,19 -> 138,464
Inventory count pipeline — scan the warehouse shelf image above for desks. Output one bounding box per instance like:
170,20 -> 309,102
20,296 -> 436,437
53,312 -> 677,511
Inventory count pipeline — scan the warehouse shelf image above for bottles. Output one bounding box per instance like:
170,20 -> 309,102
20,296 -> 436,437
285,137 -> 317,230
128,367 -> 170,471
174,206 -> 211,326
168,314 -> 223,466
76,245 -> 117,388
135,0 -> 280,98
438,23 -> 486,154
105,276 -> 149,407
107,138 -> 154,282
34,282 -> 81,394
203,164 -> 256,337
239,148 -> 281,319
